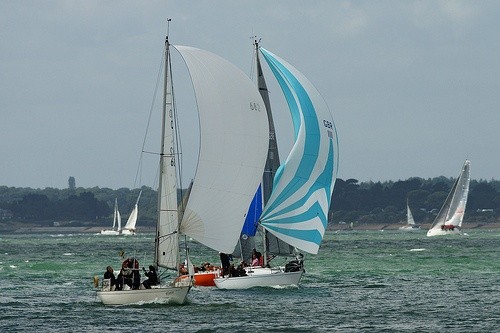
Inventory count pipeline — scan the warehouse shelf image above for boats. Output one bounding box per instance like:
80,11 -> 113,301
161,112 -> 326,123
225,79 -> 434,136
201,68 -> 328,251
176,260 -> 224,286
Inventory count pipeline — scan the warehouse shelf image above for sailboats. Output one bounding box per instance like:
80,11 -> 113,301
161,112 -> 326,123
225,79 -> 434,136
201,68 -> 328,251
426,155 -> 471,237
94,17 -> 271,307
212,33 -> 340,290
100,197 -> 123,236
121,189 -> 143,238
399,198 -> 422,231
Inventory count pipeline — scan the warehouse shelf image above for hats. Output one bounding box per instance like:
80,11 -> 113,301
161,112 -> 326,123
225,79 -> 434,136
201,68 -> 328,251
129,256 -> 133,261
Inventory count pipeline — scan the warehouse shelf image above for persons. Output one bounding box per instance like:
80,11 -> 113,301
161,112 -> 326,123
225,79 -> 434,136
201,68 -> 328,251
142,265 -> 157,289
218,247 -> 264,278
104,252 -> 141,290
179,261 -> 217,276
